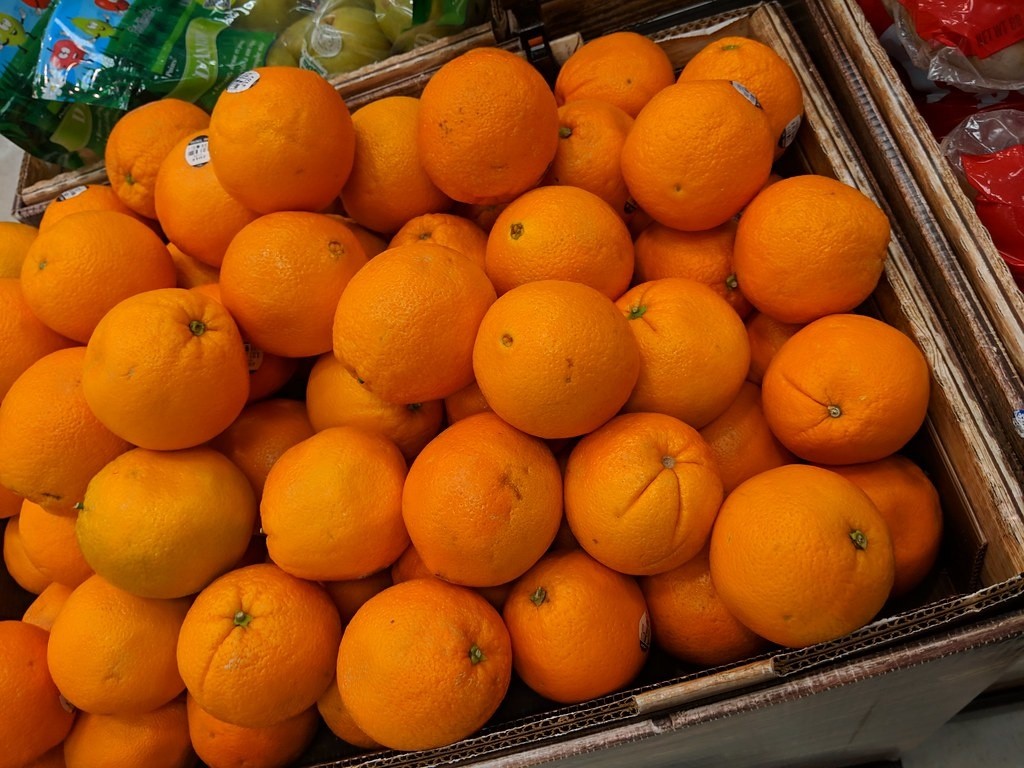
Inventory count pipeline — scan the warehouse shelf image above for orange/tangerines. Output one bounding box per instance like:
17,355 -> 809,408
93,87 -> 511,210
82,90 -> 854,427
0,35 -> 941,767
